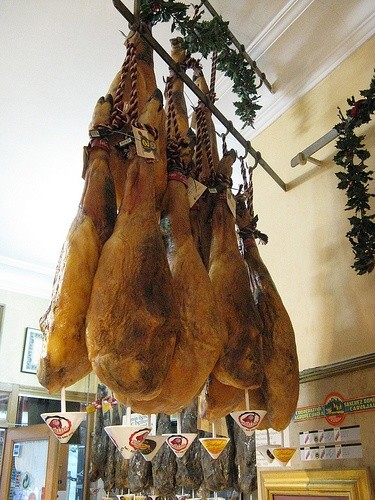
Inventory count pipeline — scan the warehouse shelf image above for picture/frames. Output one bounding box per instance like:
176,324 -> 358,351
20,327 -> 44,375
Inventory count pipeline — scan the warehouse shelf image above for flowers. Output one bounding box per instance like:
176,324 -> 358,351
333,68 -> 375,276
140,1 -> 264,130
